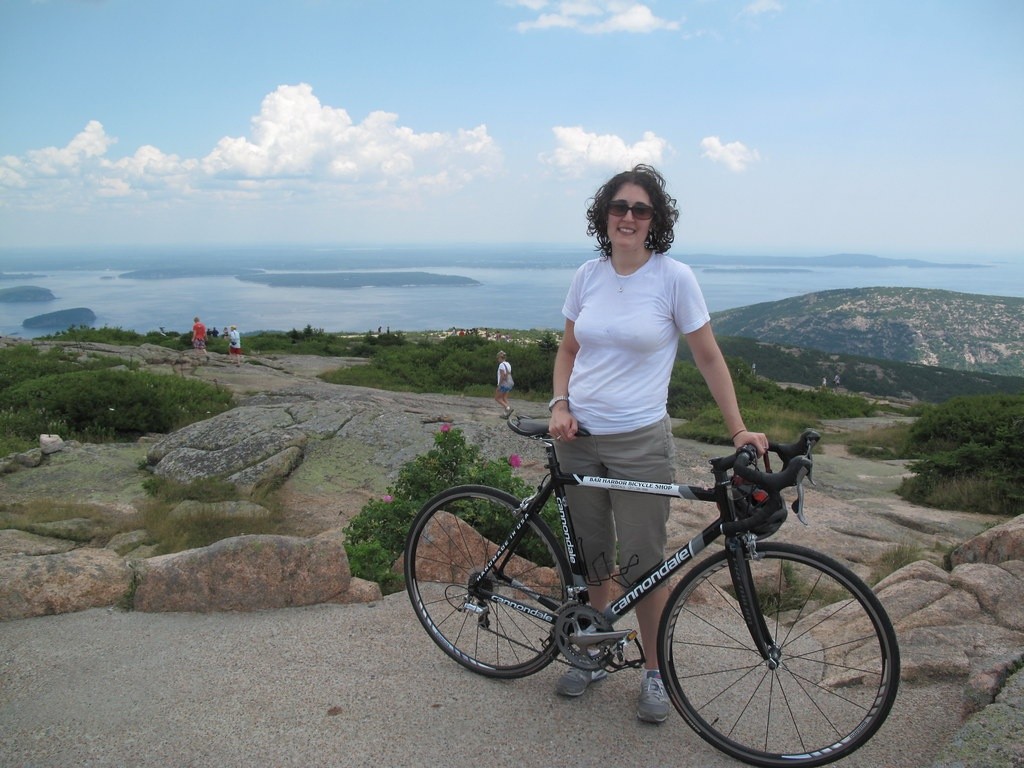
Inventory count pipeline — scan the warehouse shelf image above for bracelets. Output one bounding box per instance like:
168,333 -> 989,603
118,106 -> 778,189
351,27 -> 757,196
732,429 -> 745,439
549,395 -> 567,413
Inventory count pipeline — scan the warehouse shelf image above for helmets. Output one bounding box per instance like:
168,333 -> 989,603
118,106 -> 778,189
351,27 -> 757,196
727,472 -> 789,541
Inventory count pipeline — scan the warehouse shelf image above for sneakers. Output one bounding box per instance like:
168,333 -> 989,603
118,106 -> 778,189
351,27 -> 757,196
555,656 -> 606,696
636,666 -> 670,722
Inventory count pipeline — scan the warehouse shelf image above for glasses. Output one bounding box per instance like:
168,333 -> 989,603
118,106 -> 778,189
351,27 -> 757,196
604,200 -> 654,221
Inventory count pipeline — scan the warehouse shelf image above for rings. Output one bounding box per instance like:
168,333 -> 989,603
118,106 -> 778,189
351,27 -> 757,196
763,447 -> 767,449
557,436 -> 561,439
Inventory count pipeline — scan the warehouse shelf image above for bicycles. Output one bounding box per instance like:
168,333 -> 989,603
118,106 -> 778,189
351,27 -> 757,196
405,417 -> 900,768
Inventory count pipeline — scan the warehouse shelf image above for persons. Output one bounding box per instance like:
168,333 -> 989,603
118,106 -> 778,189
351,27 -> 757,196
223,326 -> 228,340
496,330 -> 501,342
466,328 -> 479,336
211,327 -> 219,338
822,375 -> 827,387
459,329 -> 464,336
192,317 -> 210,361
546,167 -> 769,722
495,351 -> 514,419
206,328 -> 211,338
229,326 -> 242,367
834,373 -> 840,388
485,328 -> 489,340
750,360 -> 756,376
505,333 -> 509,343
450,327 -> 458,336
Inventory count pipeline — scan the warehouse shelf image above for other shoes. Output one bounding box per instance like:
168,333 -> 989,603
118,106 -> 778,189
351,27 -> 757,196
500,413 -> 509,419
505,407 -> 514,417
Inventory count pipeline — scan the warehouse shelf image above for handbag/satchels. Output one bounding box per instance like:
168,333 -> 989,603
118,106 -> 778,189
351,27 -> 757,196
231,341 -> 236,345
499,363 -> 514,389
